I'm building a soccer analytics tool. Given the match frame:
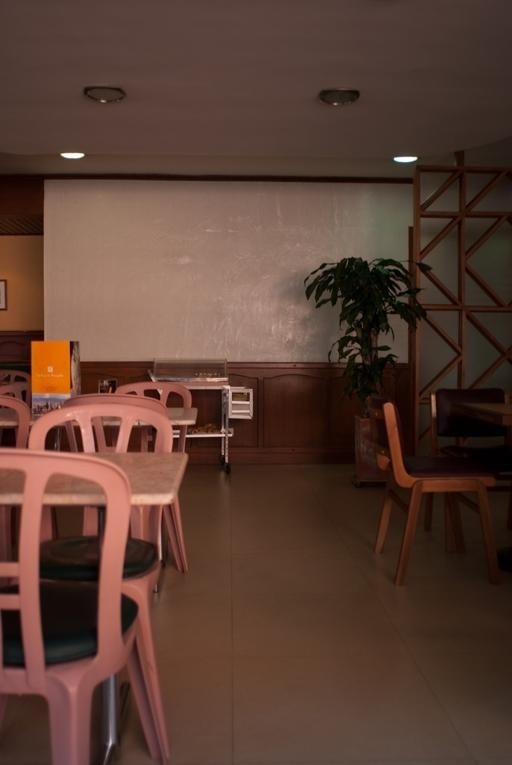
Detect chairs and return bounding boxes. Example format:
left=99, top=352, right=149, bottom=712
left=363, top=397, right=505, bottom=587
left=1, top=370, right=198, bottom=763
left=422, top=382, right=511, bottom=535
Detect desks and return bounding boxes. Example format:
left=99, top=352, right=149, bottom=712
left=435, top=398, right=512, bottom=555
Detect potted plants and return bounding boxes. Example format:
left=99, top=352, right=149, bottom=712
left=301, top=256, right=432, bottom=486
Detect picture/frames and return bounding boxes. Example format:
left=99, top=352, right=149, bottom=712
left=0, top=279, right=8, bottom=312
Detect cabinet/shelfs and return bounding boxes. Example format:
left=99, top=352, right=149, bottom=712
left=145, top=368, right=235, bottom=476
